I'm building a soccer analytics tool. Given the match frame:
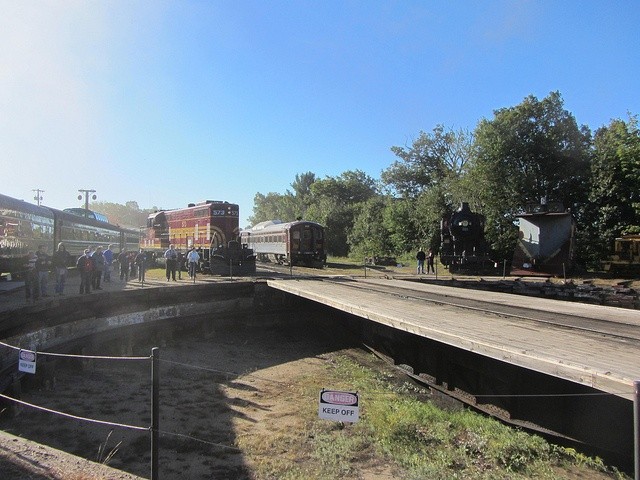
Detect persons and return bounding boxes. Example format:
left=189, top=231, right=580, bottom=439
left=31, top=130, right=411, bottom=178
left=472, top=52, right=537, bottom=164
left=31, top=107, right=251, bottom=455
left=53, top=242, right=72, bottom=296
left=136, top=249, right=147, bottom=282
left=186, top=245, right=201, bottom=280
left=426, top=248, right=435, bottom=274
left=104, top=244, right=113, bottom=283
left=36, top=245, right=52, bottom=303
left=77, top=250, right=96, bottom=294
left=22, top=249, right=41, bottom=302
left=164, top=245, right=177, bottom=281
left=117, top=247, right=129, bottom=282
left=416, top=247, right=426, bottom=274
left=172, top=247, right=183, bottom=280
left=91, top=247, right=104, bottom=291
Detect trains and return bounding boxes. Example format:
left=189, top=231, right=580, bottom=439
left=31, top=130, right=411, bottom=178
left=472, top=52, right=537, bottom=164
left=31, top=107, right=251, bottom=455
left=439, top=201, right=485, bottom=272
left=138, top=200, right=257, bottom=275
left=0, top=193, right=140, bottom=276
left=240, top=219, right=328, bottom=268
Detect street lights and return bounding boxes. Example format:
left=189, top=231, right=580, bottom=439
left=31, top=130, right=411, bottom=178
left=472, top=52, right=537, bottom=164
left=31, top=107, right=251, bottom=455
left=78, top=189, right=97, bottom=218
left=32, top=189, right=45, bottom=206
